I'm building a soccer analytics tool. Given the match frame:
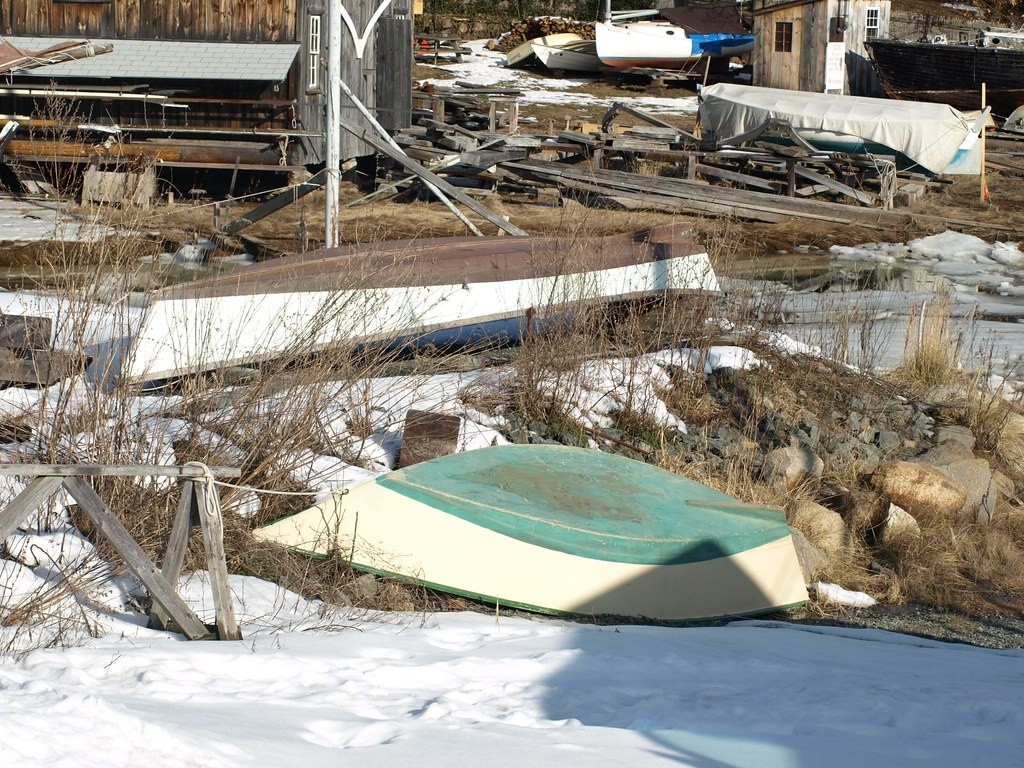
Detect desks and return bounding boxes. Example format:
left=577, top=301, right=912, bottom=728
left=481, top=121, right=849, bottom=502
left=414, top=33, right=463, bottom=65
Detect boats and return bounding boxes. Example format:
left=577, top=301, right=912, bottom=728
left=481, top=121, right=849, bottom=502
left=702, top=81, right=983, bottom=174
left=246, top=439, right=814, bottom=627
left=507, top=19, right=756, bottom=73
left=863, top=37, right=1024, bottom=109
left=112, top=222, right=727, bottom=386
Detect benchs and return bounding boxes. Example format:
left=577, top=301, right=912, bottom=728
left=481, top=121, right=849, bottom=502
left=429, top=47, right=473, bottom=54
left=413, top=48, right=440, bottom=54
